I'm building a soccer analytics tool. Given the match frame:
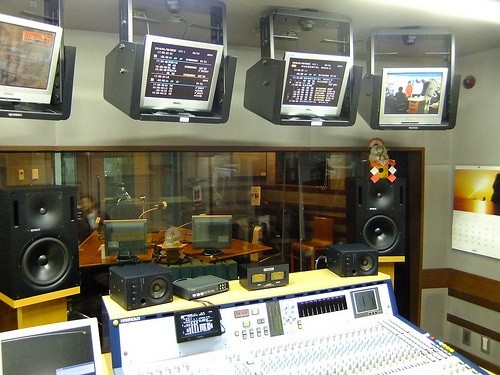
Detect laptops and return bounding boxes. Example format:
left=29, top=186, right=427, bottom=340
left=0, top=317, right=105, bottom=375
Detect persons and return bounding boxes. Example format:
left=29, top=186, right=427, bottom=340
left=405, top=80, right=413, bottom=98
left=396, top=86, right=408, bottom=111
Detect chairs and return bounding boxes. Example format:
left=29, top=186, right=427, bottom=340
left=291, top=217, right=334, bottom=271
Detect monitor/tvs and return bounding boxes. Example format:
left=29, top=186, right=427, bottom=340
left=103, top=218, right=147, bottom=262
left=0, top=13, right=63, bottom=103
left=192, top=214, right=232, bottom=255
left=378, top=67, right=449, bottom=124
left=139, top=33, right=224, bottom=112
left=279, top=51, right=351, bottom=117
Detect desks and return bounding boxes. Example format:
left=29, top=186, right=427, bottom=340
left=79, top=220, right=273, bottom=269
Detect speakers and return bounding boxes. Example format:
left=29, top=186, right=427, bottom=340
left=345, top=151, right=406, bottom=255
left=109, top=262, right=174, bottom=311
left=0, top=184, right=80, bottom=301
left=326, top=243, right=378, bottom=277
